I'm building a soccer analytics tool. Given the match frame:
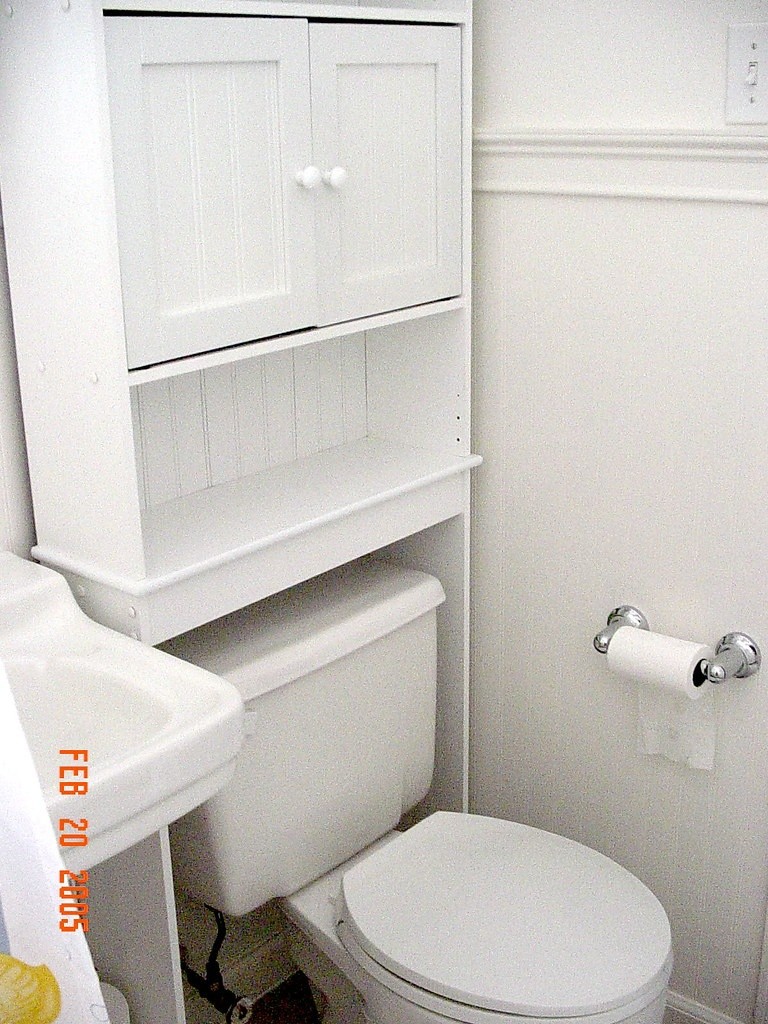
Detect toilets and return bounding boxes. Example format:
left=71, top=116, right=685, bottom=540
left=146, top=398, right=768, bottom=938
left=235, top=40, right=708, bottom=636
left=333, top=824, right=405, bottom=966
left=169, top=557, right=672, bottom=1024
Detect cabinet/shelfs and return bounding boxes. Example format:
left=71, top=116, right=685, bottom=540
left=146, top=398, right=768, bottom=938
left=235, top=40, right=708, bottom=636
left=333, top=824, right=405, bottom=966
left=0, top=0, right=472, bottom=372
left=17, top=300, right=484, bottom=1024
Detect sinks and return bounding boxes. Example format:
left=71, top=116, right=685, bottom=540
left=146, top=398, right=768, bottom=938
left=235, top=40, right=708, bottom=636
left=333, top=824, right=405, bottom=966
left=0, top=557, right=243, bottom=885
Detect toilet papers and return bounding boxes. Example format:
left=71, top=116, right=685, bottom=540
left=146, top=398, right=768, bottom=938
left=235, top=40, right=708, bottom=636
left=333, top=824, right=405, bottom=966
left=606, top=623, right=712, bottom=702
